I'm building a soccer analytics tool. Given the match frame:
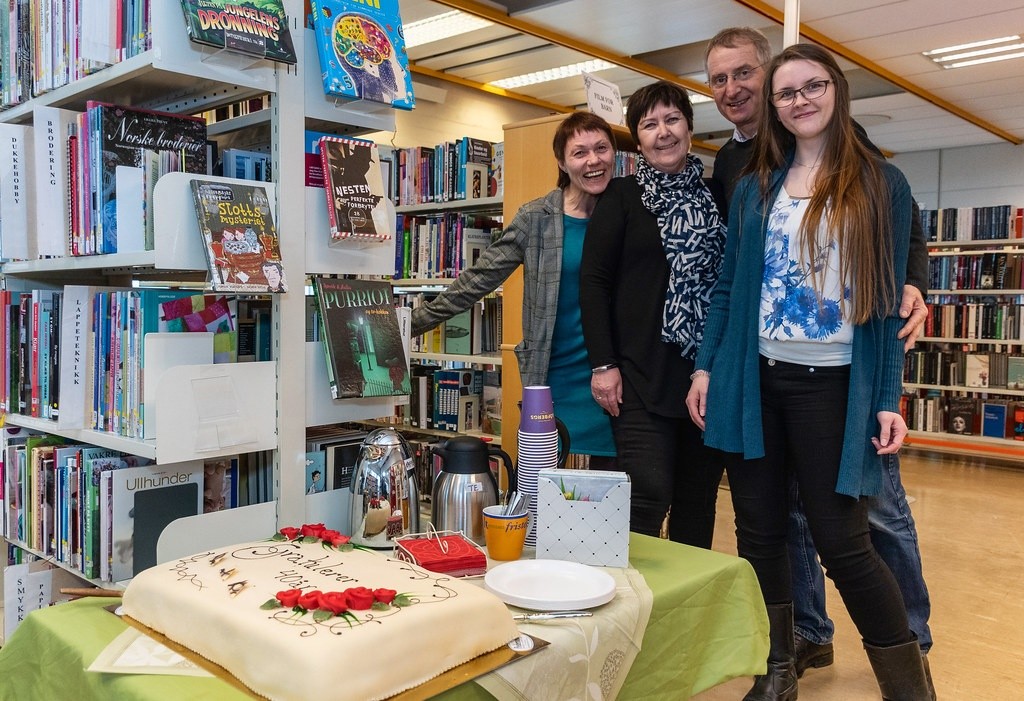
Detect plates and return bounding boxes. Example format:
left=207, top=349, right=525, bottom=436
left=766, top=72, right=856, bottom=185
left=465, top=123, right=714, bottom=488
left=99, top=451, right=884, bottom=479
left=484, top=559, right=617, bottom=611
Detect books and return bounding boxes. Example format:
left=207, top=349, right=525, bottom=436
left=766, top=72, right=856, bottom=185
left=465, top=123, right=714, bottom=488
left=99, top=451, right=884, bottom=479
left=0, top=0, right=516, bottom=657
left=886, top=198, right=1023, bottom=450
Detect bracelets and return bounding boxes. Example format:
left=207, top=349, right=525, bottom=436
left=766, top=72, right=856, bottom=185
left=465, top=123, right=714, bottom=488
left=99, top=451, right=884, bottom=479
left=592, top=364, right=617, bottom=373
left=690, top=370, right=711, bottom=380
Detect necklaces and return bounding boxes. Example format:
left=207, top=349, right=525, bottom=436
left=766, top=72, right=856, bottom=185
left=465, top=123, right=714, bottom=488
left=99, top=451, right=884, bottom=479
left=793, top=160, right=824, bottom=169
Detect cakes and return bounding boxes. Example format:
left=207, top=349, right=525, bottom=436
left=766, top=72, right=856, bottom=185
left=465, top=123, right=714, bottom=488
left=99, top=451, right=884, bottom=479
left=120, top=522, right=521, bottom=701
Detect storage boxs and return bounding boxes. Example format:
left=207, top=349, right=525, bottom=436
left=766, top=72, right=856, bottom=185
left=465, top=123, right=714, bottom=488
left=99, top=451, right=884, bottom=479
left=431, top=366, right=483, bottom=434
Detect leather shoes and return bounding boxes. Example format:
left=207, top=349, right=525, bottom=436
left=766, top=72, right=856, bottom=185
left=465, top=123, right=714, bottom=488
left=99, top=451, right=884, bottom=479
left=793, top=632, right=834, bottom=678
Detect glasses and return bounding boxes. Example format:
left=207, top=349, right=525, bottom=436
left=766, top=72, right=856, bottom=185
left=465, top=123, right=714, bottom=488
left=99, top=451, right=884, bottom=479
left=704, top=61, right=770, bottom=89
left=768, top=79, right=835, bottom=108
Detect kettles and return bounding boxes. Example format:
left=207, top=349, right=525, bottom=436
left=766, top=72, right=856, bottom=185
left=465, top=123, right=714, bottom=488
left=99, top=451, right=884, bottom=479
left=428, top=436, right=514, bottom=547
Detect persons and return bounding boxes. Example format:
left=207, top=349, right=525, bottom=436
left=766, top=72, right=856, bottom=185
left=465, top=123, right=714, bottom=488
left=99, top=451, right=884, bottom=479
left=685, top=43, right=940, bottom=701
left=705, top=27, right=930, bottom=682
left=408, top=109, right=624, bottom=492
left=580, top=81, right=734, bottom=552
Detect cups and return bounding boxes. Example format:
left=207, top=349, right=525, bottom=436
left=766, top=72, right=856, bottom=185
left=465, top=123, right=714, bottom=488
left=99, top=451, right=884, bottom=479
left=518, top=385, right=558, bottom=548
left=483, top=504, right=534, bottom=561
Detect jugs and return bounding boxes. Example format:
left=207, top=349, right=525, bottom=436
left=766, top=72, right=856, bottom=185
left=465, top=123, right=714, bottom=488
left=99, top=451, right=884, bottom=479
left=346, top=427, right=420, bottom=550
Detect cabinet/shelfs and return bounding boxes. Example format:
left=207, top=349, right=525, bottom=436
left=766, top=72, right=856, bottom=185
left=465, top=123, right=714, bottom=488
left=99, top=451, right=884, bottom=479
left=902, top=204, right=1023, bottom=466
left=351, top=193, right=503, bottom=518
left=0, top=51, right=277, bottom=591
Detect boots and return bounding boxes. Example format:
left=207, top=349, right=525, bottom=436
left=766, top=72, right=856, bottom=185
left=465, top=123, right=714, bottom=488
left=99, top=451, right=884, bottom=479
left=860, top=629, right=932, bottom=701
left=742, top=600, right=798, bottom=701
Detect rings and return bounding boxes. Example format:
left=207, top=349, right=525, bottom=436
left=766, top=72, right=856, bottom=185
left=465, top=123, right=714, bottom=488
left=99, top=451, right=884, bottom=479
left=596, top=396, right=602, bottom=399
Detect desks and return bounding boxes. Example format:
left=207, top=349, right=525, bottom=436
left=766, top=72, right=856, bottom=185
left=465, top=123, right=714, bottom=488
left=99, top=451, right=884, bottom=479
left=0, top=510, right=772, bottom=701
